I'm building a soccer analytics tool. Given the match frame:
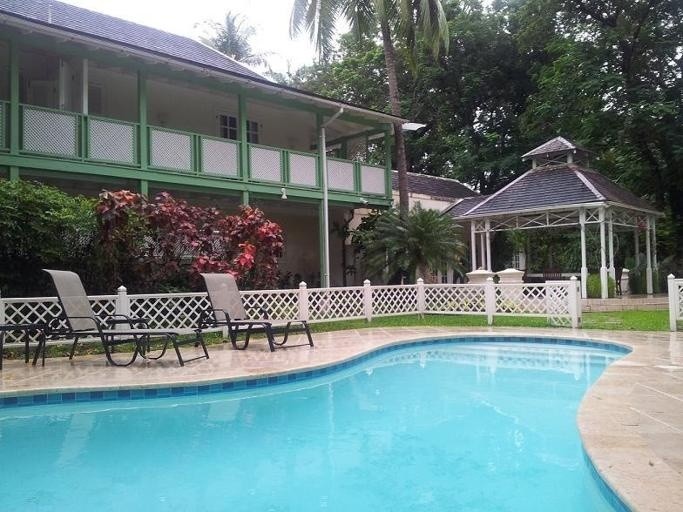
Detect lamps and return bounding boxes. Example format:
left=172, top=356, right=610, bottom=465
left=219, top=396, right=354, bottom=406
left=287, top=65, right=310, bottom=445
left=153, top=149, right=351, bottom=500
left=359, top=198, right=369, bottom=206
left=281, top=188, right=288, bottom=200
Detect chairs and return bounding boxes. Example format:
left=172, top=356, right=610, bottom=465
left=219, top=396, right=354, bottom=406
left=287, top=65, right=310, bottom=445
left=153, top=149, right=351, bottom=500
left=543, top=268, right=561, bottom=295
left=40, top=268, right=209, bottom=367
left=195, top=272, right=314, bottom=352
left=615, top=267, right=622, bottom=295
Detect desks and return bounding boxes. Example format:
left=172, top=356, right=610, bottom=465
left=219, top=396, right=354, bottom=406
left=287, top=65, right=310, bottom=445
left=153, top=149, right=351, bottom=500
left=106, top=318, right=152, bottom=356
left=0, top=323, right=46, bottom=371
